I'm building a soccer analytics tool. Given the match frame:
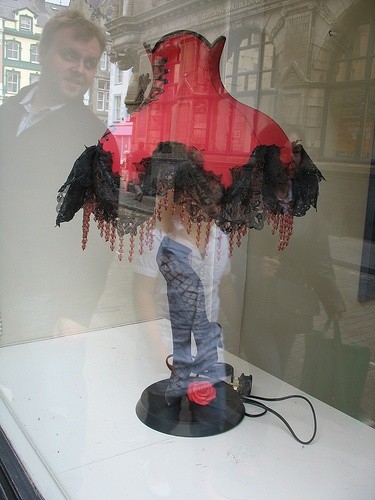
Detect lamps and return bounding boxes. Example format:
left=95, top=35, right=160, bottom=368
left=53, top=28, right=326, bottom=441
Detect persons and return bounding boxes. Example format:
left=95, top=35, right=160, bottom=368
left=218, top=120, right=346, bottom=382
left=0, top=13, right=119, bottom=345
left=135, top=160, right=230, bottom=436
left=55, top=29, right=319, bottom=408
